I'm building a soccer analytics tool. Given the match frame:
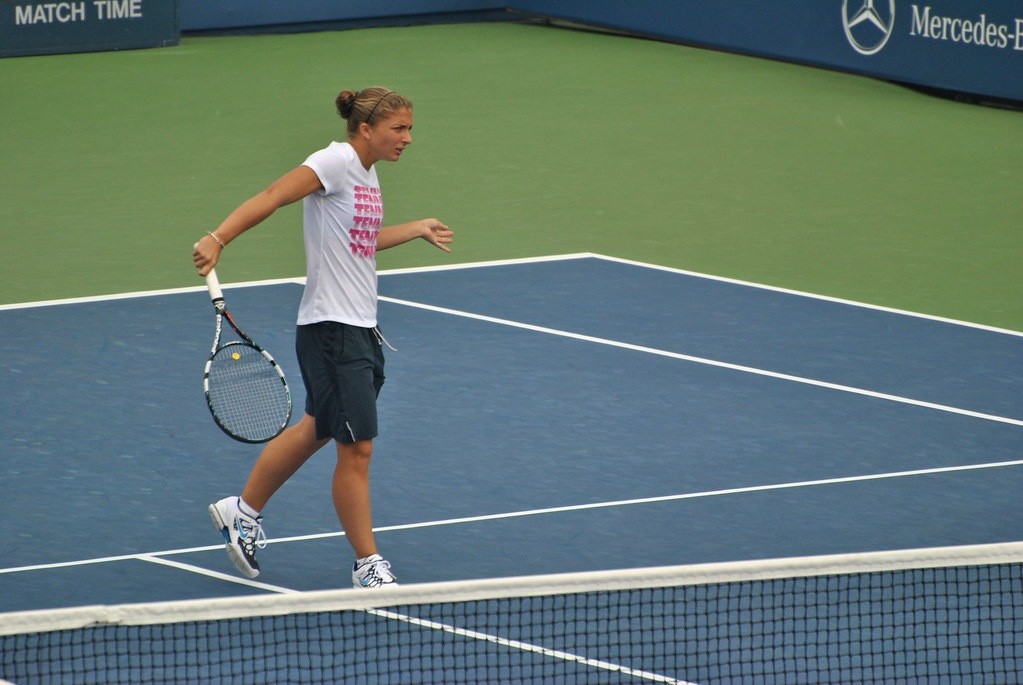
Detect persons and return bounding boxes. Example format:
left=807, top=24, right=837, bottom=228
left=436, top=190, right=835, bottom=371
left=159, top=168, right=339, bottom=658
left=192, top=87, right=455, bottom=590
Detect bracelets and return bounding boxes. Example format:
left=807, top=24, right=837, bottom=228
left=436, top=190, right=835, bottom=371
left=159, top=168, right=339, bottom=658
left=209, top=232, right=225, bottom=248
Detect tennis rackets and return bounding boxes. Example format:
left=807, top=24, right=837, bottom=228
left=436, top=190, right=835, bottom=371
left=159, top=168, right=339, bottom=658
left=193, top=241, right=293, bottom=444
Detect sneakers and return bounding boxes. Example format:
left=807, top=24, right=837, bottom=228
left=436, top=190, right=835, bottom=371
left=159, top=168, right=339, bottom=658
left=351, top=558, right=398, bottom=588
left=210, top=494, right=268, bottom=578
